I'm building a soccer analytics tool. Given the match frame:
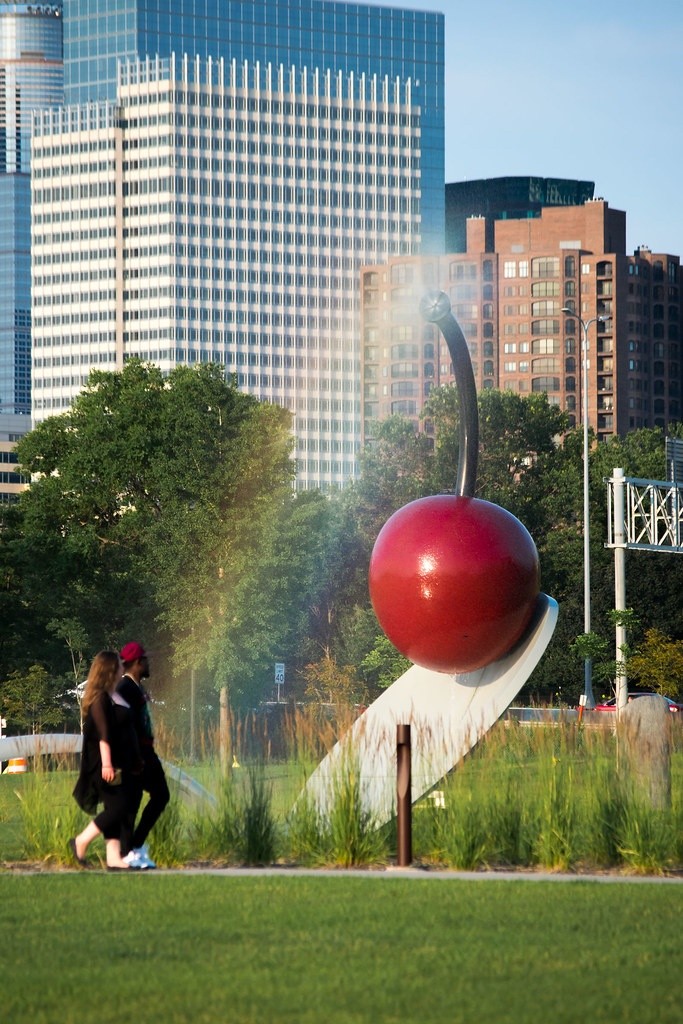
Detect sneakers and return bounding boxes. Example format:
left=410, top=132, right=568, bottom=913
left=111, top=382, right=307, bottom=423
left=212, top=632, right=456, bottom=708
left=123, top=844, right=156, bottom=869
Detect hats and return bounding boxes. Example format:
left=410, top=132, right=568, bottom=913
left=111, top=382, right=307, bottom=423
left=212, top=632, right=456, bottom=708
left=119, top=641, right=145, bottom=663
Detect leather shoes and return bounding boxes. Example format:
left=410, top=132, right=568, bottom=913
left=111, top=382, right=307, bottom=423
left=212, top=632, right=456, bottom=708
left=106, top=864, right=136, bottom=872
left=70, top=839, right=87, bottom=865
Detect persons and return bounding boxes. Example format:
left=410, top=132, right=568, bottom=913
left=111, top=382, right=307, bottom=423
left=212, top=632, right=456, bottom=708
left=111, top=642, right=170, bottom=869
left=65, top=650, right=143, bottom=872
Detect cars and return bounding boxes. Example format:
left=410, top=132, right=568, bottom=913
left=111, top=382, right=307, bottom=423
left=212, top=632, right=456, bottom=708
left=594, top=692, right=683, bottom=715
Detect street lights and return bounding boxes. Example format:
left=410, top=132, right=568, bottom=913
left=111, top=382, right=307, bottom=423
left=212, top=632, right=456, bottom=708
left=562, top=306, right=609, bottom=704
